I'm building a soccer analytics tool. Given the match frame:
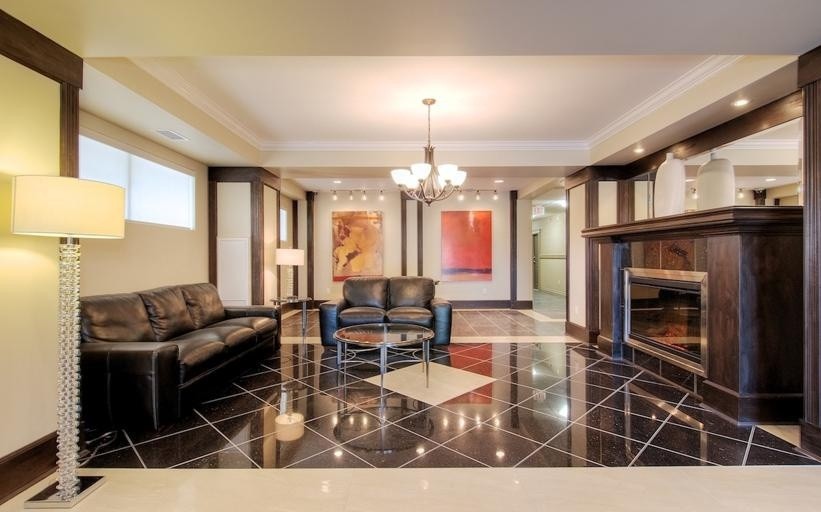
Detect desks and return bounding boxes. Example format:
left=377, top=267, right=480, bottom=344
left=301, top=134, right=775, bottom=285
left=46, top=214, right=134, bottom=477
left=270, top=296, right=313, bottom=331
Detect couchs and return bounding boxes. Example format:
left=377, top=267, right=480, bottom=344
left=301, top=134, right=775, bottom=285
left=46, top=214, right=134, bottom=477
left=319, top=276, right=454, bottom=348
left=79, top=282, right=282, bottom=435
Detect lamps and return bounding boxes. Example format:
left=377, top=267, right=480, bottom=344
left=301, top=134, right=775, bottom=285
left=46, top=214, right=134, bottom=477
left=9, top=173, right=127, bottom=509
left=276, top=248, right=306, bottom=300
left=274, top=385, right=307, bottom=443
left=390, top=98, right=467, bottom=209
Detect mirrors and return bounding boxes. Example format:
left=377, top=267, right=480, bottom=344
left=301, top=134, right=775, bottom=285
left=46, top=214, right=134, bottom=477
left=208, top=166, right=282, bottom=306
left=565, top=165, right=621, bottom=345
left=626, top=114, right=805, bottom=223
